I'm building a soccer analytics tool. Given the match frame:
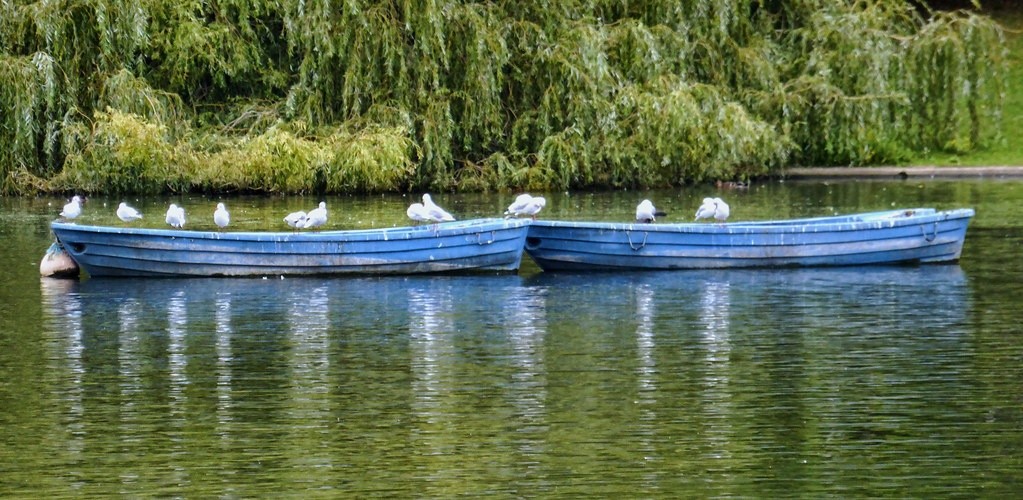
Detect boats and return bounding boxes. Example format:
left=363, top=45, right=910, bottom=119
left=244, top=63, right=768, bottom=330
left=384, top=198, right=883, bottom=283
left=51, top=216, right=529, bottom=277
left=521, top=205, right=978, bottom=271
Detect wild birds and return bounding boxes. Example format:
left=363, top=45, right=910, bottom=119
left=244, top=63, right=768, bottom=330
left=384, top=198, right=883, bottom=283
left=636, top=199, right=667, bottom=223
left=165, top=204, right=185, bottom=230
left=116, top=202, right=142, bottom=223
left=283, top=201, right=328, bottom=234
left=213, top=203, right=229, bottom=233
left=406, top=193, right=456, bottom=230
left=694, top=197, right=730, bottom=223
left=58, top=195, right=85, bottom=219
left=504, top=194, right=546, bottom=221
